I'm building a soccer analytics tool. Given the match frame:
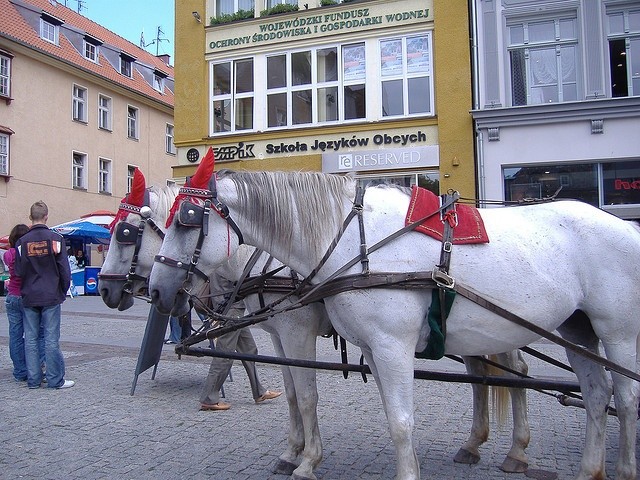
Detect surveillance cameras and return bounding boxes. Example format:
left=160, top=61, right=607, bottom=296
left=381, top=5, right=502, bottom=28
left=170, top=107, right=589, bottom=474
left=192, top=11, right=201, bottom=23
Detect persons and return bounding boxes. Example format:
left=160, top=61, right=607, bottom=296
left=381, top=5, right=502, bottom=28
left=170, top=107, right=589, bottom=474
left=3, top=224, right=49, bottom=383
left=198, top=272, right=282, bottom=410
left=14, top=201, right=75, bottom=388
left=75, top=250, right=88, bottom=267
left=67, top=248, right=79, bottom=270
left=164, top=314, right=191, bottom=345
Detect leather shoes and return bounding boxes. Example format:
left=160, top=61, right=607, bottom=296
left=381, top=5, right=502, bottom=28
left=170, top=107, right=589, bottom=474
left=201, top=401, right=232, bottom=410
left=254, top=391, right=283, bottom=403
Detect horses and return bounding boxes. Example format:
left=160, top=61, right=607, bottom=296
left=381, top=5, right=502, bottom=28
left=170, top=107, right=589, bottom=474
left=147, top=144, right=640, bottom=479
left=92, top=168, right=535, bottom=474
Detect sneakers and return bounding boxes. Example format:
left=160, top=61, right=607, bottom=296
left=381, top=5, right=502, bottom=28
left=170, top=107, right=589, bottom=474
left=48, top=380, right=75, bottom=389
left=165, top=339, right=178, bottom=344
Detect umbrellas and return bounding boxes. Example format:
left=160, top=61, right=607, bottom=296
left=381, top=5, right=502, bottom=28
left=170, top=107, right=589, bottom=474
left=75, top=216, right=116, bottom=261
left=0, top=236, right=9, bottom=243
left=80, top=210, right=117, bottom=219
left=49, top=218, right=112, bottom=252
